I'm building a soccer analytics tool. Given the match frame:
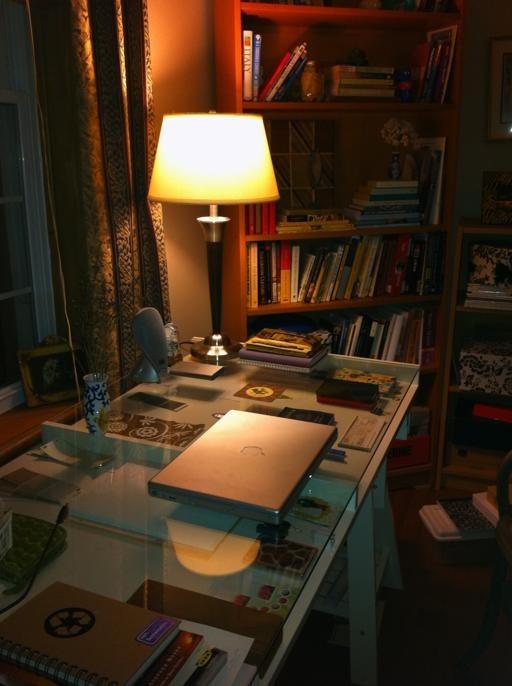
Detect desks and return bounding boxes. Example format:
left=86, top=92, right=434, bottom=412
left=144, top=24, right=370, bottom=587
left=0, top=342, right=421, bottom=685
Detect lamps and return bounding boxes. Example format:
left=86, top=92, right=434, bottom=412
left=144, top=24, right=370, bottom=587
left=147, top=109, right=280, bottom=365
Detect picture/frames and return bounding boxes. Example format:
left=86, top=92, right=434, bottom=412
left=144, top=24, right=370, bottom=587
left=17, top=342, right=91, bottom=408
left=487, top=36, right=512, bottom=142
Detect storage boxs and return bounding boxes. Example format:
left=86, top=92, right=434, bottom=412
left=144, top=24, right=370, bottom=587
left=476, top=170, right=512, bottom=224
left=447, top=391, right=511, bottom=451
left=459, top=334, right=512, bottom=397
left=470, top=244, right=510, bottom=289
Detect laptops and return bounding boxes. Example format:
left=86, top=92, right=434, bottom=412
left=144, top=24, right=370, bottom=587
left=149, top=410, right=338, bottom=527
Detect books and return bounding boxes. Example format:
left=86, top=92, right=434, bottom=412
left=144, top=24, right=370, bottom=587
left=246, top=234, right=420, bottom=307
left=243, top=30, right=452, bottom=105
left=0, top=467, right=81, bottom=505
left=360, top=0, right=449, bottom=13
left=186, top=648, right=228, bottom=686
left=464, top=245, right=512, bottom=311
left=169, top=360, right=225, bottom=381
left=240, top=310, right=409, bottom=373
left=1, top=579, right=182, bottom=686
left=246, top=180, right=423, bottom=236
left=261, top=0, right=332, bottom=7
left=1, top=626, right=207, bottom=686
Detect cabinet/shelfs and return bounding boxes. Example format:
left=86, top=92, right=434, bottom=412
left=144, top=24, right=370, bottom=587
left=212, top=1, right=466, bottom=491
left=433, top=217, right=512, bottom=496
